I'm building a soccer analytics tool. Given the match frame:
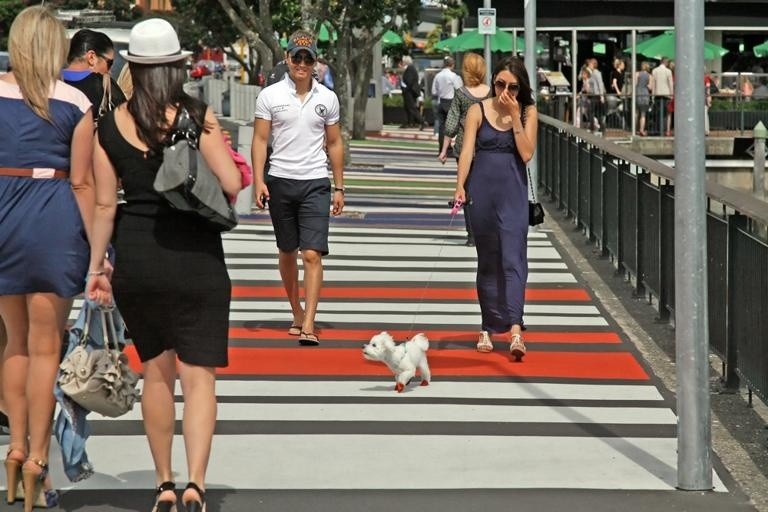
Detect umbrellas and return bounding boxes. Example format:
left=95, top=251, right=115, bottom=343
left=432, top=27, right=544, bottom=54
left=753, top=40, right=768, bottom=58
left=279, top=23, right=403, bottom=49
left=623, top=31, right=730, bottom=62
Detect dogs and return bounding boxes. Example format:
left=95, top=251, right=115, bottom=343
left=360, top=331, right=432, bottom=393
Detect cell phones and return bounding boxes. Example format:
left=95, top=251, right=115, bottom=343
left=262, top=197, right=266, bottom=205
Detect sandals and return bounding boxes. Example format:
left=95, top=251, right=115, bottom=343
left=509, top=334, right=526, bottom=361
left=476, top=330, right=494, bottom=353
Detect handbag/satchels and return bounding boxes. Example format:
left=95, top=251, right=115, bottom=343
left=527, top=200, right=545, bottom=226
left=58, top=305, right=141, bottom=418
left=153, top=103, right=238, bottom=232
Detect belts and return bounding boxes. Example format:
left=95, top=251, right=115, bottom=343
left=0, top=168, right=69, bottom=178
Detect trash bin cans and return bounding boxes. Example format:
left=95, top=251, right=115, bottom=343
left=347, top=80, right=383, bottom=135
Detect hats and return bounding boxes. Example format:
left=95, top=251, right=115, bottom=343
left=118, top=17, right=195, bottom=65
left=287, top=30, right=318, bottom=61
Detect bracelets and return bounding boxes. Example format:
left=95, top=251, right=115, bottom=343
left=335, top=188, right=344, bottom=193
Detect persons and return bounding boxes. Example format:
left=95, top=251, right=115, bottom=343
left=453, top=57, right=538, bottom=362
left=704, top=66, right=754, bottom=137
left=431, top=53, right=495, bottom=247
left=251, top=29, right=346, bottom=348
left=382, top=55, right=428, bottom=128
left=578, top=54, right=676, bottom=137
left=0, top=4, right=242, bottom=512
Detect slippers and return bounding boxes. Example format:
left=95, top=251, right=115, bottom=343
left=287, top=325, right=301, bottom=336
left=299, top=332, right=320, bottom=345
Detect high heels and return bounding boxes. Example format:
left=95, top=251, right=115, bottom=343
left=22, top=457, right=59, bottom=512
left=182, top=483, right=207, bottom=512
left=150, top=481, right=180, bottom=512
left=5, top=445, right=28, bottom=503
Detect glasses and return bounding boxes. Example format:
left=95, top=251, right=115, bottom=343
left=290, top=54, right=315, bottom=66
left=100, top=54, right=114, bottom=70
left=494, top=81, right=519, bottom=93
